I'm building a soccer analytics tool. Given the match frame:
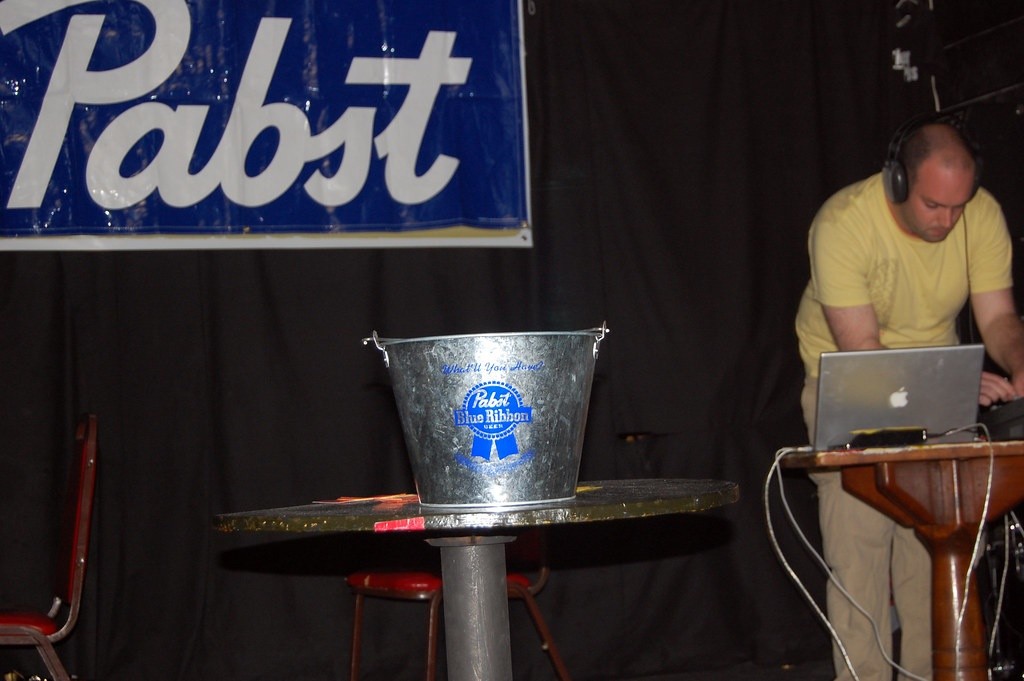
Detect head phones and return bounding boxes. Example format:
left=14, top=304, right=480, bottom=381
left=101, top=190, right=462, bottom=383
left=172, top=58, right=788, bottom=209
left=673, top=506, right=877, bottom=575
left=885, top=111, right=981, bottom=203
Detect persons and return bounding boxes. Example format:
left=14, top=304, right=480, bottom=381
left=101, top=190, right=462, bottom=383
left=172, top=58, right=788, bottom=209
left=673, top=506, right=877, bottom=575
left=794, top=120, right=1024, bottom=681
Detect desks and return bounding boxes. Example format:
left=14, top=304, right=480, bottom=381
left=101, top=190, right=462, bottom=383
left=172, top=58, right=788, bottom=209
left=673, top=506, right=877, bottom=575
left=214, top=479, right=740, bottom=681
left=782, top=440, right=1024, bottom=680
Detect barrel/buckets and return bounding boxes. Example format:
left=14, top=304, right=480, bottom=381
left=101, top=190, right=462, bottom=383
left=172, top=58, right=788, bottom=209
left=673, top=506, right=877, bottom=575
left=361, top=322, right=612, bottom=509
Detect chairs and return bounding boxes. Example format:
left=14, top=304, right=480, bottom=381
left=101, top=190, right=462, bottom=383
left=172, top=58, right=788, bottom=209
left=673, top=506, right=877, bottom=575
left=347, top=523, right=571, bottom=681
left=0, top=411, right=98, bottom=680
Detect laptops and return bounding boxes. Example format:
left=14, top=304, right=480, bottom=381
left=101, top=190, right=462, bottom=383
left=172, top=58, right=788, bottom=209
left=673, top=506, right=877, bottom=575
left=811, top=343, right=985, bottom=452
